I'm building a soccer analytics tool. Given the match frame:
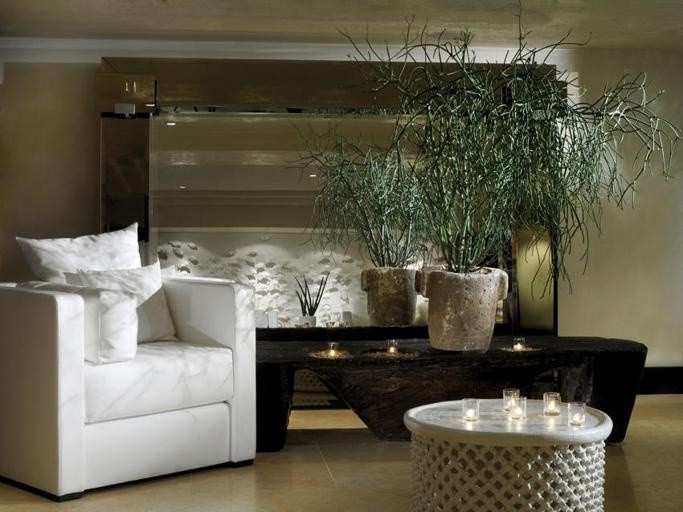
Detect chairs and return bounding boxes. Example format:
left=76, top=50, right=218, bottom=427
left=0, top=266, right=259, bottom=503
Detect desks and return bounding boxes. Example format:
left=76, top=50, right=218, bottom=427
left=256, top=336, right=648, bottom=454
left=399, top=396, right=613, bottom=510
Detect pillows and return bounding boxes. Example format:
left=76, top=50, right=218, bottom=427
left=16, top=222, right=142, bottom=297
left=65, top=258, right=183, bottom=343
left=16, top=280, right=137, bottom=363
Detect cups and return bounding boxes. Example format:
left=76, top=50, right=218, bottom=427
left=512, top=337, right=525, bottom=350
left=542, top=392, right=562, bottom=415
left=462, top=398, right=481, bottom=421
left=268, top=310, right=277, bottom=328
left=501, top=387, right=527, bottom=420
left=566, top=401, right=586, bottom=426
left=387, top=339, right=400, bottom=354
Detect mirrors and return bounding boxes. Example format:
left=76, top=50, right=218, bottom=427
left=146, top=105, right=522, bottom=328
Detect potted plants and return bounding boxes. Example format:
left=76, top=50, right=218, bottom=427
left=297, top=118, right=430, bottom=325
left=304, top=16, right=682, bottom=350
left=291, top=264, right=329, bottom=327
